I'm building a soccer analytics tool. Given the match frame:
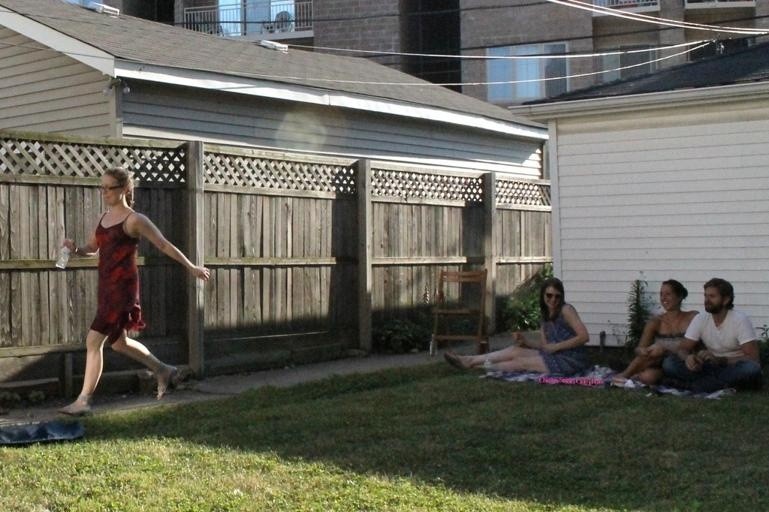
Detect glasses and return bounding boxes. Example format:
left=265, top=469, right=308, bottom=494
left=99, top=185, right=123, bottom=192
left=546, top=293, right=561, bottom=299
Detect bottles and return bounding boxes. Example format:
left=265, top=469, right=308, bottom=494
left=592, top=364, right=605, bottom=393
left=55, top=246, right=70, bottom=269
left=430, top=334, right=438, bottom=359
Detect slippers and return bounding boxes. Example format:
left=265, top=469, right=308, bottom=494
left=444, top=352, right=471, bottom=370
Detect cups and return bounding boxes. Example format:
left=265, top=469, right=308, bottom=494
left=480, top=341, right=489, bottom=355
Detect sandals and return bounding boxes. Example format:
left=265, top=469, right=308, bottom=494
left=58, top=395, right=91, bottom=417
left=155, top=362, right=178, bottom=401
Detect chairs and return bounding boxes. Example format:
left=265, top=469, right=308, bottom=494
left=429, top=269, right=488, bottom=356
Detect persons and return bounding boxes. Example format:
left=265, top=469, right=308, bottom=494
left=659, top=277, right=764, bottom=395
left=608, top=277, right=699, bottom=388
left=59, top=166, right=210, bottom=415
left=445, top=277, right=594, bottom=377
